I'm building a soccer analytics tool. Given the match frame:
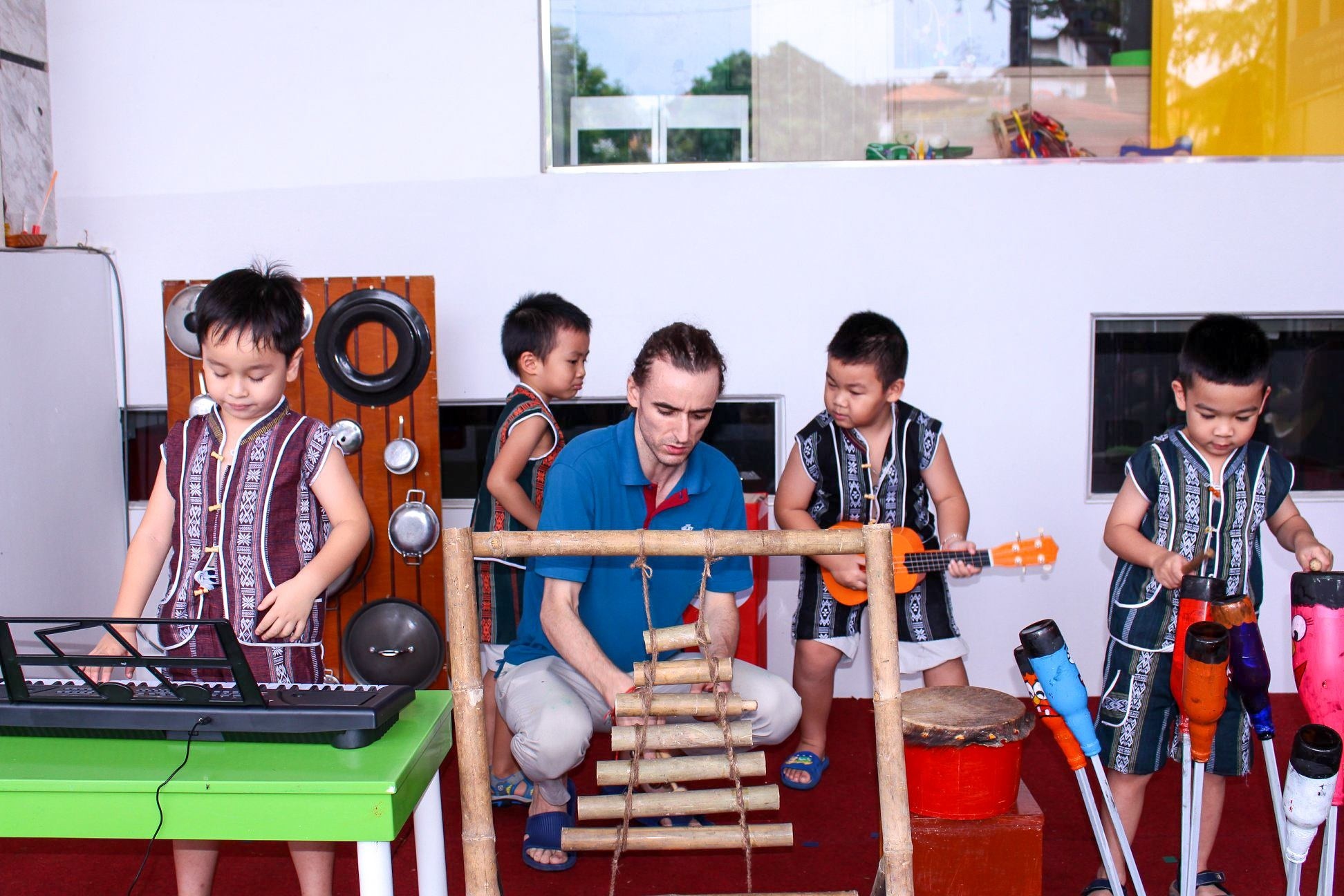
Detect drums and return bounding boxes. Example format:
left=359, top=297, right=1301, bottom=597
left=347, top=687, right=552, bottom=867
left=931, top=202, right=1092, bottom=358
left=902, top=685, right=1036, bottom=819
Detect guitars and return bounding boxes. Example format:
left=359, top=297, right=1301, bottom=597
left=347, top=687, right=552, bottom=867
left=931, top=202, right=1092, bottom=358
left=820, top=520, right=1059, bottom=606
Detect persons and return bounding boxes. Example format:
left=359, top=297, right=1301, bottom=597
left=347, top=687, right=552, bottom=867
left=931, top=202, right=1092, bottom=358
left=76, top=267, right=372, bottom=895
left=1077, top=312, right=1333, bottom=896
left=493, top=321, right=803, bottom=872
left=774, top=310, right=985, bottom=789
left=467, top=292, right=594, bottom=806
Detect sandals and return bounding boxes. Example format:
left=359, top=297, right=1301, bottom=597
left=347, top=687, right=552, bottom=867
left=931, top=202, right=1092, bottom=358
left=1081, top=879, right=1128, bottom=896
left=489, top=770, right=535, bottom=807
left=1168, top=870, right=1235, bottom=896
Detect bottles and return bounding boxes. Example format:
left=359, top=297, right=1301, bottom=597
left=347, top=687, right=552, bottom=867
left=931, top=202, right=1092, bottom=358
left=1290, top=571, right=1344, bottom=806
left=1019, top=618, right=1102, bottom=757
left=1014, top=644, right=1088, bottom=771
left=1182, top=623, right=1229, bottom=763
left=1212, top=596, right=1274, bottom=742
left=1282, top=724, right=1343, bottom=866
left=1170, top=575, right=1227, bottom=733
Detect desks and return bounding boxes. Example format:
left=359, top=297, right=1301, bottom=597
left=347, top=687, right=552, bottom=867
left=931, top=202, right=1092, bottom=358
left=0, top=689, right=456, bottom=896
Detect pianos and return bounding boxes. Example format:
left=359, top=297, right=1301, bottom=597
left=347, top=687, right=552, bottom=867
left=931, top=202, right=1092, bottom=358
left=0, top=677, right=416, bottom=751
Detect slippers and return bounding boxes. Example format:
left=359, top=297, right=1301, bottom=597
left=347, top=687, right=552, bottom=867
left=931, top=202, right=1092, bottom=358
left=780, top=751, right=829, bottom=790
left=521, top=779, right=577, bottom=871
left=604, top=785, right=714, bottom=827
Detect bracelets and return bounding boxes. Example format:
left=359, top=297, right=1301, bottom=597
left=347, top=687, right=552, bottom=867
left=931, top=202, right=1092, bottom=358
left=942, top=534, right=962, bottom=542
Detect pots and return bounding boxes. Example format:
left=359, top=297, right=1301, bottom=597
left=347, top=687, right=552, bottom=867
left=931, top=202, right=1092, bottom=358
left=383, top=414, right=420, bottom=475
left=387, top=489, right=439, bottom=565
